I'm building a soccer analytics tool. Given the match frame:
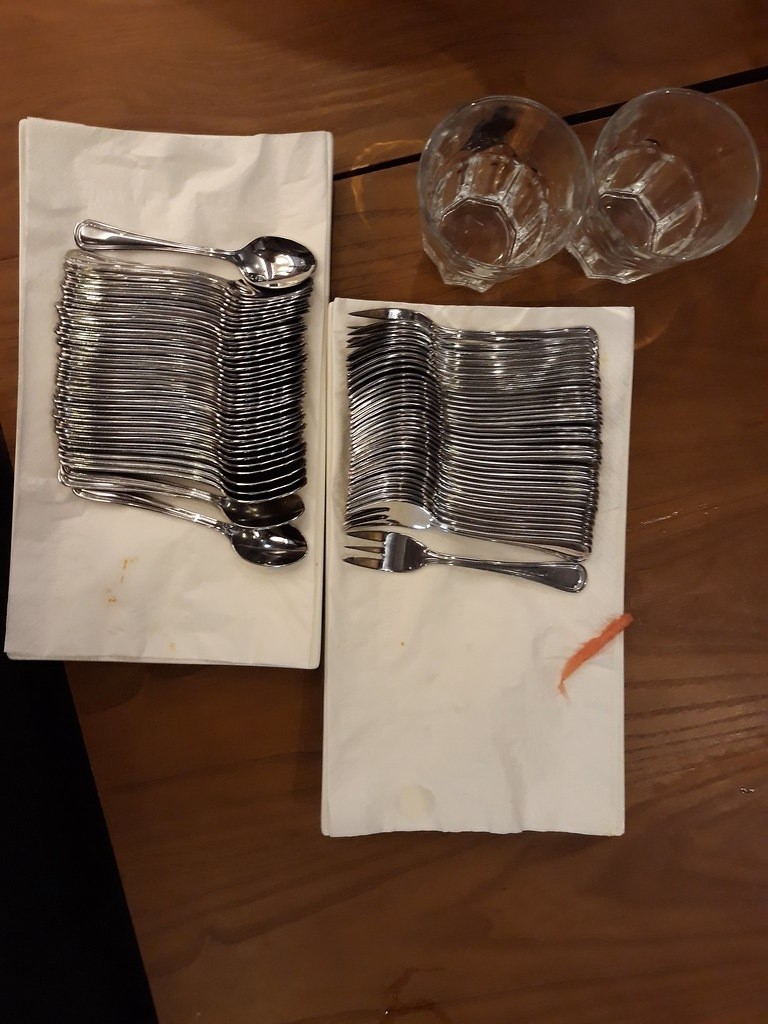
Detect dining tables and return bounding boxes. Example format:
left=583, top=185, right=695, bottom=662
left=0, top=0, right=768, bottom=1017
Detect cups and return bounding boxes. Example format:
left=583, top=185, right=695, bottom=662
left=420, top=92, right=590, bottom=295
left=564, top=87, right=762, bottom=285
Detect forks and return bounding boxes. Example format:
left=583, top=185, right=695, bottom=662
left=330, top=298, right=609, bottom=601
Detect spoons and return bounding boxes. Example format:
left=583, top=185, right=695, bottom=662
left=50, top=217, right=314, bottom=569
